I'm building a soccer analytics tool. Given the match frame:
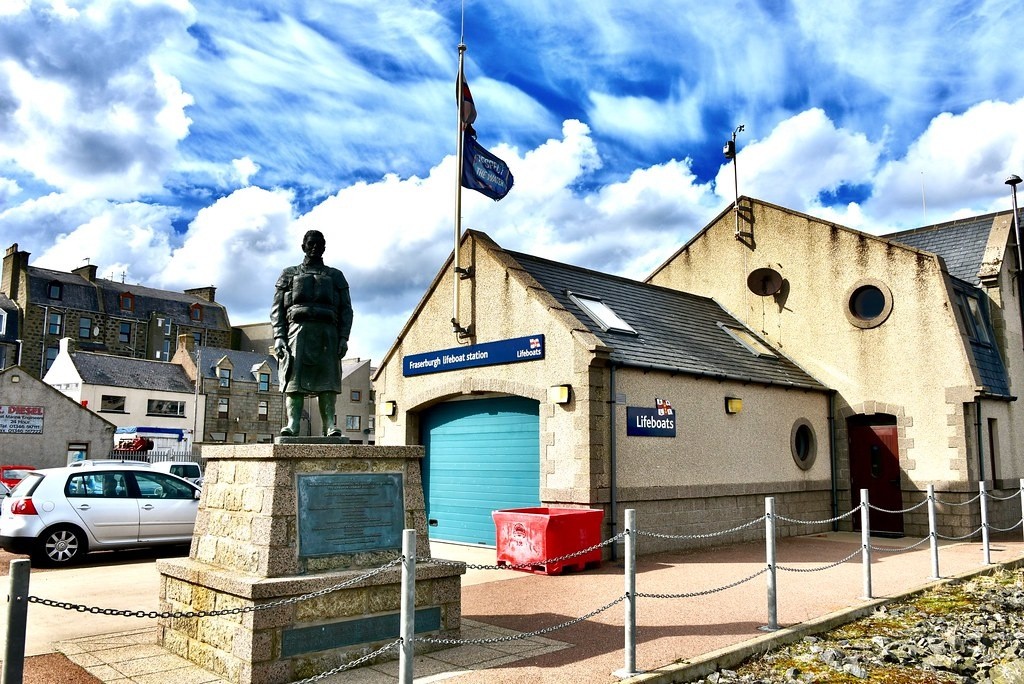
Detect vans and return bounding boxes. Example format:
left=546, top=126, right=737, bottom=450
left=64, top=457, right=203, bottom=497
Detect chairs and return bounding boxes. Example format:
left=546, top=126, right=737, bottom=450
left=175, top=469, right=181, bottom=476
left=118, top=478, right=126, bottom=497
left=103, top=479, right=118, bottom=497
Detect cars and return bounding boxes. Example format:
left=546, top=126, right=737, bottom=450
left=0, top=465, right=202, bottom=568
left=0, top=465, right=36, bottom=488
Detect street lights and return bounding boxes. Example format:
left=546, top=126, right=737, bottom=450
left=1003, top=172, right=1024, bottom=272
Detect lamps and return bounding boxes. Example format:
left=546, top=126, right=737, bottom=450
left=11, top=375, right=20, bottom=383
left=380, top=401, right=397, bottom=417
left=724, top=396, right=743, bottom=415
left=550, top=384, right=572, bottom=406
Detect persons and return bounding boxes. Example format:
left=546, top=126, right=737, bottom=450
left=269, top=230, right=354, bottom=437
